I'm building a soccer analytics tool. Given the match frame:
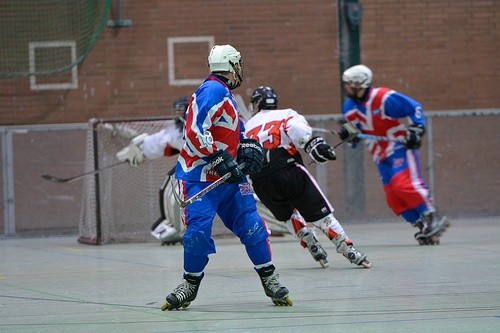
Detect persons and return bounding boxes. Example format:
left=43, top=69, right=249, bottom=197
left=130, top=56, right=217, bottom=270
left=116, top=98, right=190, bottom=241
left=338, top=65, right=447, bottom=239
left=242, top=85, right=364, bottom=264
left=165, top=45, right=289, bottom=306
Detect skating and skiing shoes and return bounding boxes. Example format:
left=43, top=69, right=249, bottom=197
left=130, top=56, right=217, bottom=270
left=336, top=238, right=372, bottom=268
left=160, top=272, right=205, bottom=310
left=303, top=232, right=330, bottom=268
left=412, top=209, right=450, bottom=245
left=253, top=265, right=294, bottom=307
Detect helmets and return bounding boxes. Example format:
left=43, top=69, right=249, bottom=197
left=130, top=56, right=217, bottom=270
left=342, top=64, right=375, bottom=89
left=171, top=96, right=190, bottom=121
left=206, top=44, right=245, bottom=90
left=251, top=85, right=279, bottom=111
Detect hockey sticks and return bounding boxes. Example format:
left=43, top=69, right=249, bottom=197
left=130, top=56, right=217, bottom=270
left=305, top=119, right=360, bottom=167
left=312, top=126, right=397, bottom=142
left=171, top=162, right=246, bottom=208
left=41, top=159, right=129, bottom=183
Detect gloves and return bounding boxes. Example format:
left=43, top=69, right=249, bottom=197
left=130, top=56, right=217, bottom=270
left=237, top=138, right=266, bottom=174
left=404, top=124, right=426, bottom=151
left=303, top=135, right=337, bottom=165
left=209, top=149, right=247, bottom=184
left=335, top=118, right=358, bottom=142
